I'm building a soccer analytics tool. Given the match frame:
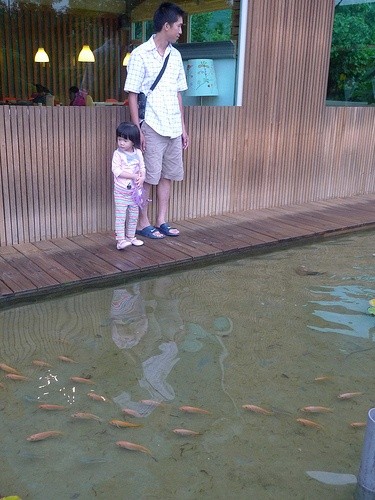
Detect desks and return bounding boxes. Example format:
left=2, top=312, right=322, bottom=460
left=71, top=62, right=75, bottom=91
left=92, top=101, right=125, bottom=106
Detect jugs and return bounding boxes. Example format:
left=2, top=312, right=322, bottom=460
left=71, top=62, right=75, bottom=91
left=45, top=93, right=55, bottom=106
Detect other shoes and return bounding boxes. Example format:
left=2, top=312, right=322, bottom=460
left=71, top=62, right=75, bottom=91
left=126, top=237, right=144, bottom=246
left=116, top=240, right=132, bottom=250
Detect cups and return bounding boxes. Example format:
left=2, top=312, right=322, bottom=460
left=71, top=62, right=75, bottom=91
left=38, top=103, right=42, bottom=106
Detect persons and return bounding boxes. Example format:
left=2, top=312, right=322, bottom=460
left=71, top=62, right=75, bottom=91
left=109, top=283, right=148, bottom=381
left=124, top=3, right=188, bottom=240
left=33, top=85, right=95, bottom=106
left=111, top=121, right=146, bottom=250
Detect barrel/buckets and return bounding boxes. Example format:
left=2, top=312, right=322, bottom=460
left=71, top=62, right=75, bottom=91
left=356, top=407, right=375, bottom=500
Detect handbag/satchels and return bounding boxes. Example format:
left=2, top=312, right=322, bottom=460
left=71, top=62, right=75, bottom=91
left=137, top=92, right=147, bottom=119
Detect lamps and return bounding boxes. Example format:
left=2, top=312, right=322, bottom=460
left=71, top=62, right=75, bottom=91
left=34, top=47, right=49, bottom=63
left=122, top=50, right=132, bottom=66
left=186, top=57, right=221, bottom=107
left=77, top=0, right=96, bottom=63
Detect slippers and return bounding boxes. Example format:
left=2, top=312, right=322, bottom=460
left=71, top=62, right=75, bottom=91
left=136, top=225, right=165, bottom=239
left=152, top=223, right=180, bottom=236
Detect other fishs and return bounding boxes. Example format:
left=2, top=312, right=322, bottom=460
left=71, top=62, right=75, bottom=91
left=0, top=354, right=368, bottom=470
